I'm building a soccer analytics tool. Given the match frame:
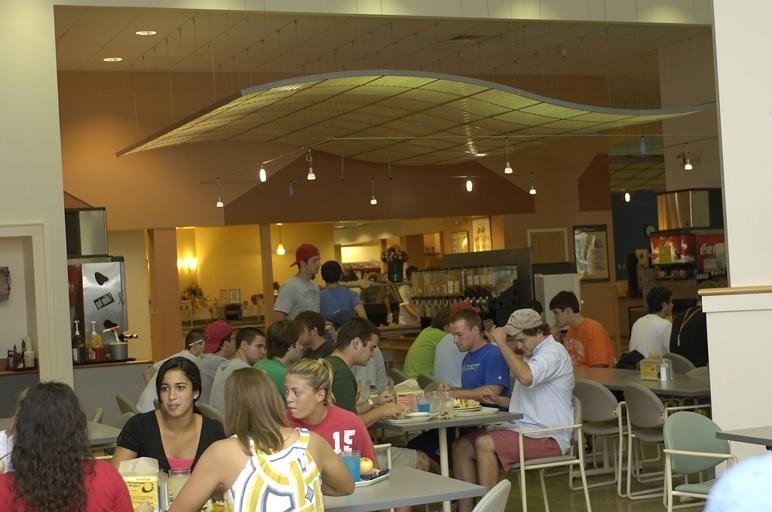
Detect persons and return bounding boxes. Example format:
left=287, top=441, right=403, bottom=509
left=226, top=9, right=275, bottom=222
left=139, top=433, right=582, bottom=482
left=548, top=290, right=616, bottom=454
left=110, top=357, right=228, bottom=473
left=628, top=286, right=674, bottom=358
left=274, top=243, right=321, bottom=327
left=314, top=259, right=370, bottom=325
left=670, top=280, right=722, bottom=419
left=0, top=385, right=33, bottom=474
left=397, top=265, right=422, bottom=326
left=0, top=381, right=155, bottom=511
left=361, top=270, right=396, bottom=326
left=404, top=308, right=510, bottom=512
left=135, top=307, right=468, bottom=512
left=452, top=307, right=575, bottom=512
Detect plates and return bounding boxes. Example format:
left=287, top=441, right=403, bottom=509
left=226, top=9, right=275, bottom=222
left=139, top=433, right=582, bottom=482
left=355, top=468, right=391, bottom=487
left=405, top=413, right=437, bottom=422
left=454, top=406, right=500, bottom=415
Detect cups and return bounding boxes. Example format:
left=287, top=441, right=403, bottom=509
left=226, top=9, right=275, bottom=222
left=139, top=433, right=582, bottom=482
left=339, top=450, right=360, bottom=482
left=416, top=395, right=430, bottom=413
left=442, top=391, right=455, bottom=409
left=168, top=467, right=191, bottom=502
left=428, top=391, right=442, bottom=412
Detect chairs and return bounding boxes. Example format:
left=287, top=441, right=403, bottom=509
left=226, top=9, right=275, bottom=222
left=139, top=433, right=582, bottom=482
left=507, top=395, right=594, bottom=510
left=569, top=381, right=664, bottom=500
left=196, top=402, right=229, bottom=433
left=113, top=413, right=137, bottom=430
left=90, top=406, right=109, bottom=423
left=662, top=409, right=738, bottom=511
left=370, top=442, right=431, bottom=511
left=662, top=350, right=699, bottom=413
left=623, top=382, right=704, bottom=502
left=471, top=477, right=512, bottom=512
left=115, top=392, right=146, bottom=412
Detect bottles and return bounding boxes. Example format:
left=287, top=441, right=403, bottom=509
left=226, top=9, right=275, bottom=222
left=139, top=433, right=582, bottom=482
left=6, top=340, right=26, bottom=370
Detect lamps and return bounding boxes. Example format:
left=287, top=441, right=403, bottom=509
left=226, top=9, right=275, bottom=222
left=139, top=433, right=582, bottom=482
left=368, top=178, right=378, bottom=205
left=677, top=138, right=693, bottom=173
left=637, top=134, right=647, bottom=157
left=502, top=135, right=512, bottom=176
left=305, top=146, right=317, bottom=182
left=276, top=222, right=286, bottom=255
left=259, top=160, right=268, bottom=182
left=286, top=178, right=296, bottom=196
left=465, top=176, right=474, bottom=192
left=623, top=183, right=633, bottom=209
left=527, top=171, right=538, bottom=202
left=215, top=178, right=224, bottom=209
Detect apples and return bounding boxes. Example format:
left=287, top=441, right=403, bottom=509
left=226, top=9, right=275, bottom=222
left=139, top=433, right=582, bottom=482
left=360, top=457, right=373, bottom=476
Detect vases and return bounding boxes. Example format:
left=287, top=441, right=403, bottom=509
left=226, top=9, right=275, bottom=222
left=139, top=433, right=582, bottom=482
left=386, top=263, right=404, bottom=283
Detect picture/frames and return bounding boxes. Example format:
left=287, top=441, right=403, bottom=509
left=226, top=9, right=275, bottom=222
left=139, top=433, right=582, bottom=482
left=228, top=286, right=241, bottom=304
left=449, top=231, right=470, bottom=254
left=572, top=223, right=610, bottom=285
left=471, top=216, right=492, bottom=253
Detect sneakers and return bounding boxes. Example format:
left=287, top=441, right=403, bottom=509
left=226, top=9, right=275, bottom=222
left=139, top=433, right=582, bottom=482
left=584, top=434, right=592, bottom=453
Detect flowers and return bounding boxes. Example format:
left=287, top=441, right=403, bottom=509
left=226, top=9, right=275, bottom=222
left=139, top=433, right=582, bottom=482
left=380, top=242, right=408, bottom=263
left=405, top=265, right=419, bottom=276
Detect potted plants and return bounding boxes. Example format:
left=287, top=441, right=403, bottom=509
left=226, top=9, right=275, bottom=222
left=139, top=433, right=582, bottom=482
left=361, top=282, right=389, bottom=326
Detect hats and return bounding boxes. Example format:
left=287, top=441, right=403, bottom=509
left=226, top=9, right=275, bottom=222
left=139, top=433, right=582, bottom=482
left=203, top=321, right=232, bottom=353
left=503, top=308, right=543, bottom=336
left=327, top=308, right=358, bottom=328
left=450, top=302, right=481, bottom=315
left=699, top=280, right=719, bottom=288
left=289, top=244, right=319, bottom=267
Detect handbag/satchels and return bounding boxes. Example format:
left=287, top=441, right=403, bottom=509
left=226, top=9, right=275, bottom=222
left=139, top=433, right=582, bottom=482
left=611, top=350, right=646, bottom=402
